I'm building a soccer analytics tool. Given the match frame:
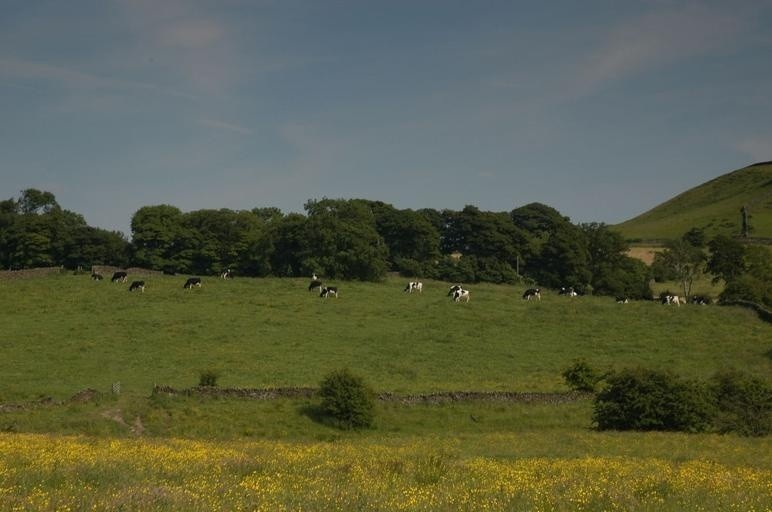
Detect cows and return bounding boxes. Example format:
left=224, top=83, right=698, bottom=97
left=184, top=278, right=201, bottom=289
left=523, top=288, right=541, bottom=302
left=447, top=285, right=470, bottom=303
left=320, top=286, right=338, bottom=298
left=111, top=272, right=128, bottom=283
left=558, top=286, right=583, bottom=297
left=404, top=282, right=423, bottom=293
left=91, top=274, right=103, bottom=280
left=220, top=269, right=231, bottom=280
left=129, top=280, right=144, bottom=292
left=662, top=294, right=709, bottom=307
left=308, top=275, right=322, bottom=292
left=616, top=296, right=628, bottom=304
